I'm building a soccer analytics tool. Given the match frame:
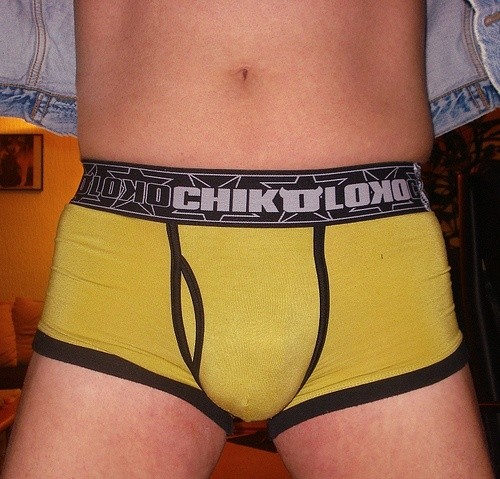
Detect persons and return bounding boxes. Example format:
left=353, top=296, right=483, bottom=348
left=3, top=2, right=500, bottom=476
